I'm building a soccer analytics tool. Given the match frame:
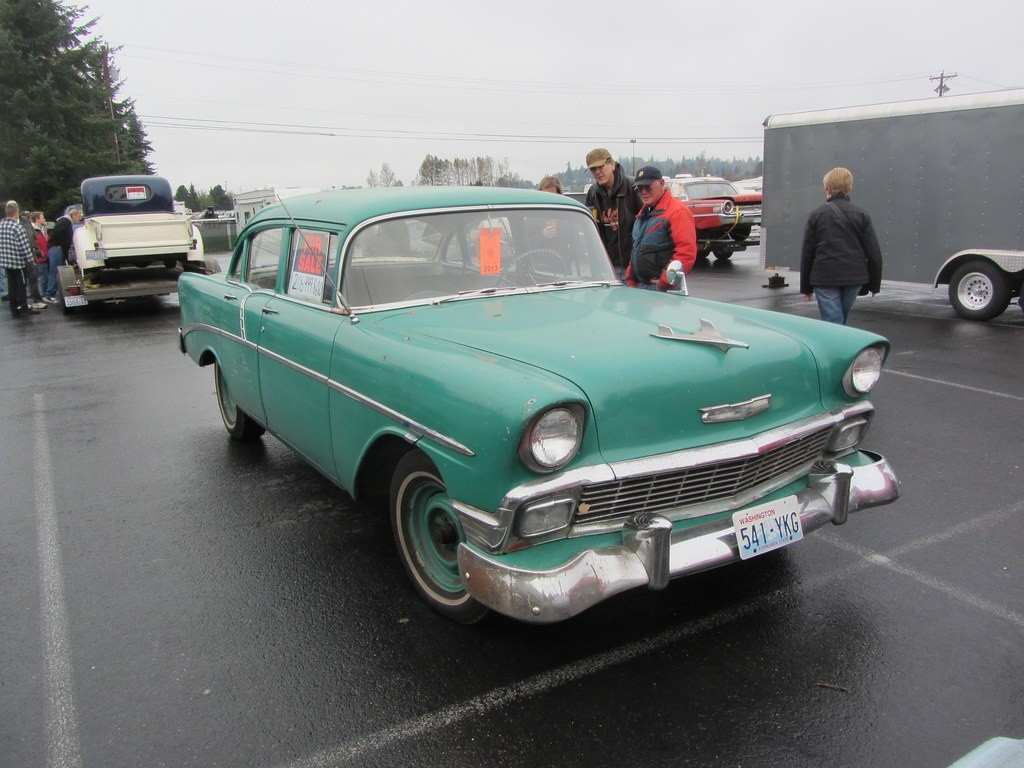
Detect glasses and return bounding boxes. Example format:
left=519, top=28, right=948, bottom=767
left=634, top=183, right=659, bottom=191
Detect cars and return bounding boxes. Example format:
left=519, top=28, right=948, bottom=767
left=64, top=174, right=204, bottom=286
left=176, top=185, right=908, bottom=634
left=223, top=172, right=762, bottom=273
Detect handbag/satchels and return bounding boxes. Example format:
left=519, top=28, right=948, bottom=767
left=858, top=284, right=869, bottom=295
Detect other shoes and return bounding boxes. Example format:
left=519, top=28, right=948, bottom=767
left=32, top=303, right=47, bottom=308
left=42, top=297, right=57, bottom=304
left=17, top=305, right=32, bottom=309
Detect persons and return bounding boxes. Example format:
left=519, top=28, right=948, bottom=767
left=584, top=149, right=644, bottom=286
left=626, top=167, right=697, bottom=292
left=799, top=167, right=882, bottom=326
left=539, top=176, right=563, bottom=195
left=0, top=201, right=83, bottom=320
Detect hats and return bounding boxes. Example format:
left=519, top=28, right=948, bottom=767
left=584, top=148, right=611, bottom=172
left=631, top=166, right=662, bottom=187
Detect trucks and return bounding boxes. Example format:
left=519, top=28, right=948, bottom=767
left=757, top=84, right=1024, bottom=326
